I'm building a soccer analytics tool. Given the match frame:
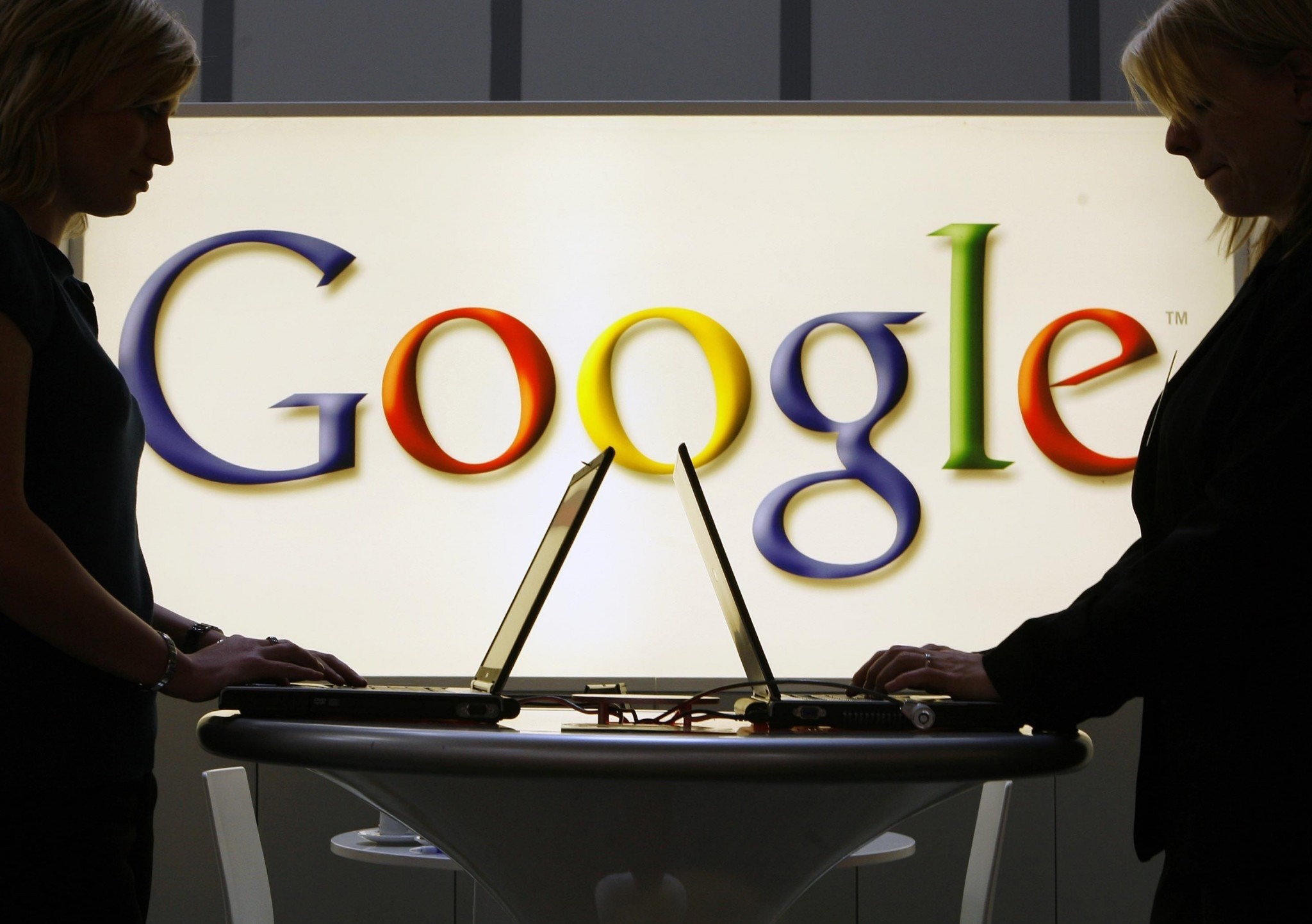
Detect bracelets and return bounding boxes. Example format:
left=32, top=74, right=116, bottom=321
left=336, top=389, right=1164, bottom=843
left=180, top=623, right=221, bottom=656
left=139, top=630, right=178, bottom=691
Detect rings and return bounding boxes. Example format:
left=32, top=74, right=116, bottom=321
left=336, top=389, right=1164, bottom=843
left=924, top=652, right=934, bottom=668
left=266, top=636, right=278, bottom=645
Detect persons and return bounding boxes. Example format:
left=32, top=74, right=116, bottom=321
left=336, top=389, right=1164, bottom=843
left=845, top=1, right=1312, bottom=924
left=0, top=1, right=369, bottom=923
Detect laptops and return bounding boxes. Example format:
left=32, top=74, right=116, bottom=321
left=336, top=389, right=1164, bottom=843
left=672, top=442, right=1023, bottom=731
left=217, top=446, right=616, bottom=723
